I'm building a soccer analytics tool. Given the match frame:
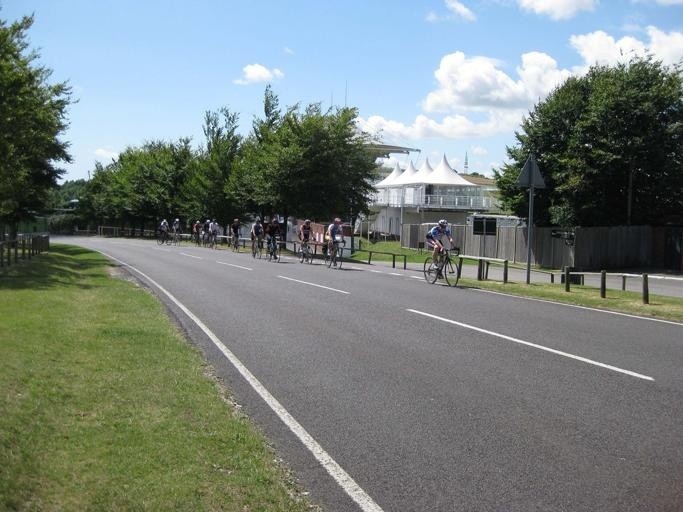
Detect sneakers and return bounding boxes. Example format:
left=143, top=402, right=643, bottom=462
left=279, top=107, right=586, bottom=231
left=431, top=263, right=438, bottom=270
left=438, top=274, right=442, bottom=278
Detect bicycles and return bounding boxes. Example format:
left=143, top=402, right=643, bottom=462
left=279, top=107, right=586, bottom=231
left=325, top=240, right=345, bottom=269
left=423, top=248, right=460, bottom=287
left=252, top=232, right=262, bottom=259
left=266, top=234, right=280, bottom=263
left=193, top=232, right=220, bottom=250
left=157, top=229, right=181, bottom=247
left=298, top=239, right=314, bottom=264
left=230, top=234, right=242, bottom=253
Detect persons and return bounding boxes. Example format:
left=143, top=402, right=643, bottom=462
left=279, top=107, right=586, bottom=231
left=160, top=216, right=282, bottom=259
left=324, top=217, right=345, bottom=266
left=299, top=220, right=315, bottom=252
left=426, top=219, right=453, bottom=279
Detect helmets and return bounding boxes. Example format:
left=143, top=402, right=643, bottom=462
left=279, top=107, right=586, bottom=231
left=439, top=219, right=447, bottom=225
